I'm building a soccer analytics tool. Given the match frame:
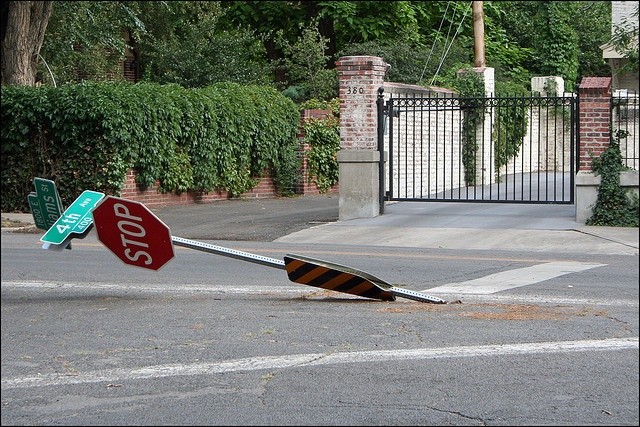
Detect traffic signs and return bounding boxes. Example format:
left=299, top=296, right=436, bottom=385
left=40, top=190, right=105, bottom=246
left=28, top=177, right=72, bottom=250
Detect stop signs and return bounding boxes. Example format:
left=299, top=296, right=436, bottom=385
left=92, top=195, right=175, bottom=271
